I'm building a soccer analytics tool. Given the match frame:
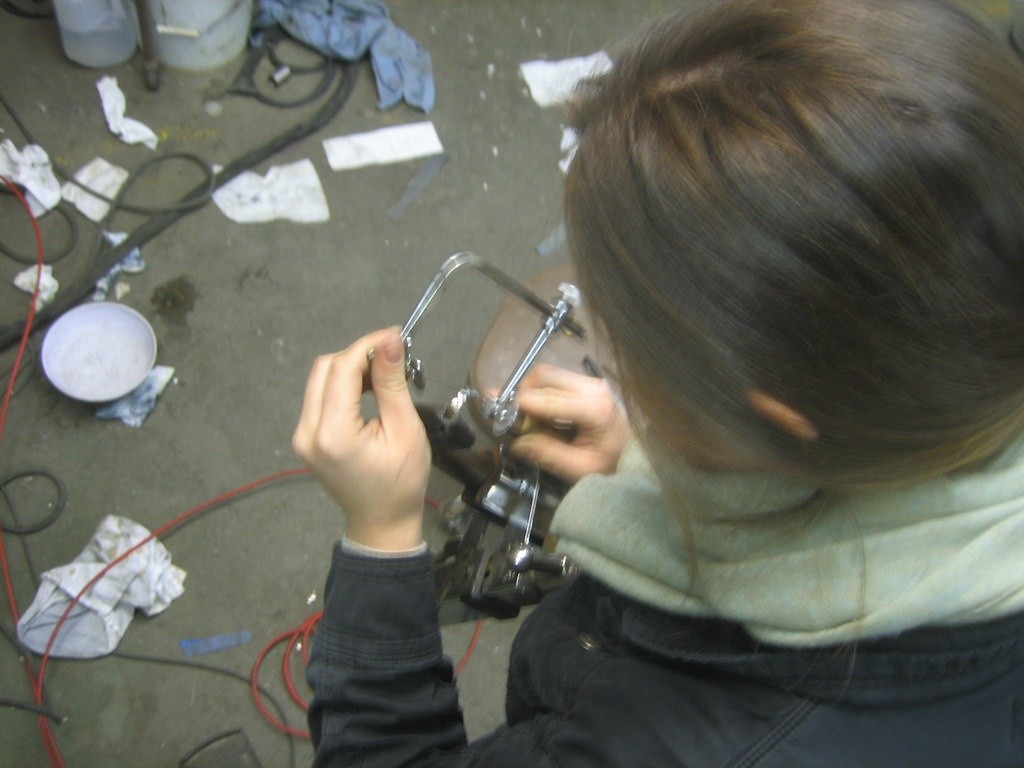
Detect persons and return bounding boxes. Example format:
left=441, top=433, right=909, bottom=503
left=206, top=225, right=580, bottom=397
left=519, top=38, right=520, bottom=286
left=292, top=1, right=1024, bottom=768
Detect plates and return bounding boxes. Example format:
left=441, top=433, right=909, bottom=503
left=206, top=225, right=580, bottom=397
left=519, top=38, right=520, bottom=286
left=43, top=301, right=158, bottom=403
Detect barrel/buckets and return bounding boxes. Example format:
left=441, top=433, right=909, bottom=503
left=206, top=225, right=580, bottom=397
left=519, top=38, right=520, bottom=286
left=141, top=0, right=253, bottom=71
left=54, top=0, right=145, bottom=67
